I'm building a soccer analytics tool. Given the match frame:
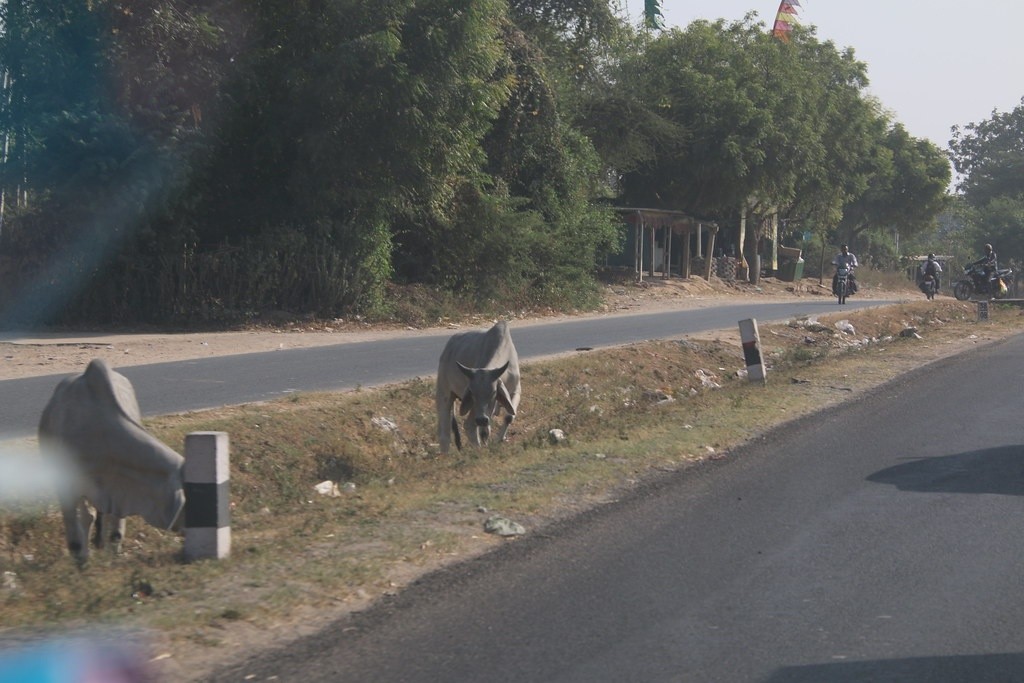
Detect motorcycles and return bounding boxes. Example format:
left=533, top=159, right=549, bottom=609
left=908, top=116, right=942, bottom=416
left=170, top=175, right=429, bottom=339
left=920, top=269, right=942, bottom=301
left=831, top=261, right=858, bottom=305
left=954, top=255, right=1016, bottom=300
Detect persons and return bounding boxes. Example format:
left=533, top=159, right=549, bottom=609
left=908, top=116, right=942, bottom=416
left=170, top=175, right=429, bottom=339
left=831, top=244, right=858, bottom=294
left=919, top=253, right=942, bottom=292
left=972, top=244, right=998, bottom=301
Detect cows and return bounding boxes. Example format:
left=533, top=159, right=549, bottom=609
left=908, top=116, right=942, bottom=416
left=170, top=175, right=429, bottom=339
left=37, top=356, right=189, bottom=572
left=434, top=320, right=522, bottom=459
